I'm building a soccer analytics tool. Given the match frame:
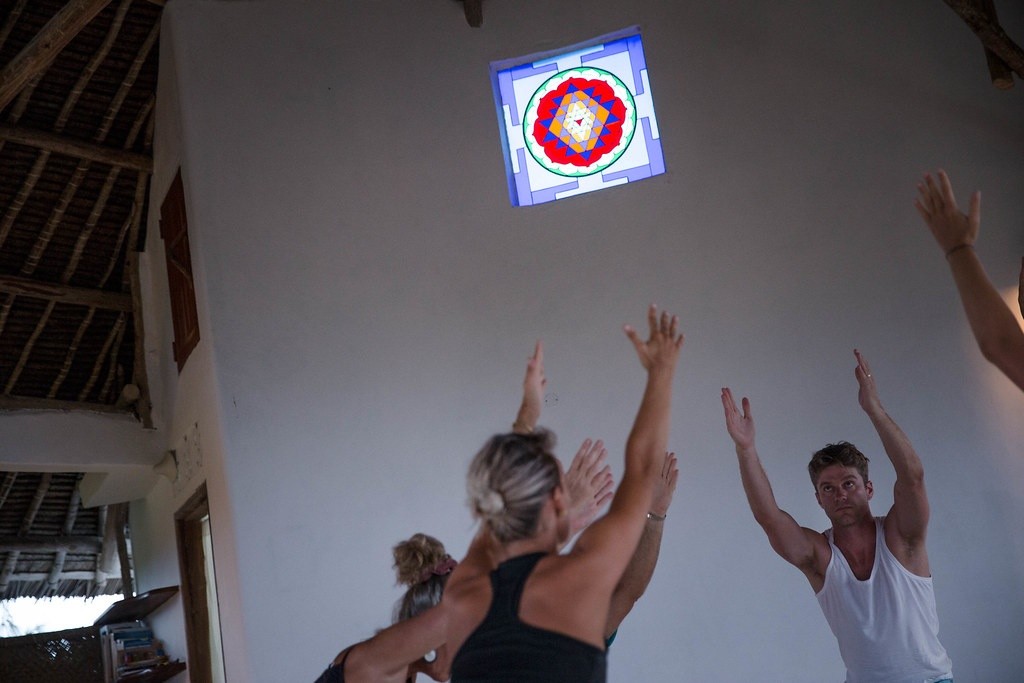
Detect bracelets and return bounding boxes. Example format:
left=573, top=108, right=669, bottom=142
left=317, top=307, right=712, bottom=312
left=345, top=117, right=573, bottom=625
left=945, top=244, right=975, bottom=260
left=647, top=513, right=666, bottom=521
left=512, top=422, right=533, bottom=433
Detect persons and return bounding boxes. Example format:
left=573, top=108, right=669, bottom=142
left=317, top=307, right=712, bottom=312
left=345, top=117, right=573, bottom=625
left=443, top=302, right=685, bottom=683
left=722, top=349, right=953, bottom=683
left=313, top=438, right=679, bottom=683
left=913, top=169, right=1024, bottom=391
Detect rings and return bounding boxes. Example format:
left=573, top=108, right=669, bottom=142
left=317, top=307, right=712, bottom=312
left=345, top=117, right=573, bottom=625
left=868, top=375, right=870, bottom=377
left=663, top=330, right=670, bottom=335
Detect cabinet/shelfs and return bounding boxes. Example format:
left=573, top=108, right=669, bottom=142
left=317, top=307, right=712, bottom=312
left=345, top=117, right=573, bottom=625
left=94, top=585, right=188, bottom=683
